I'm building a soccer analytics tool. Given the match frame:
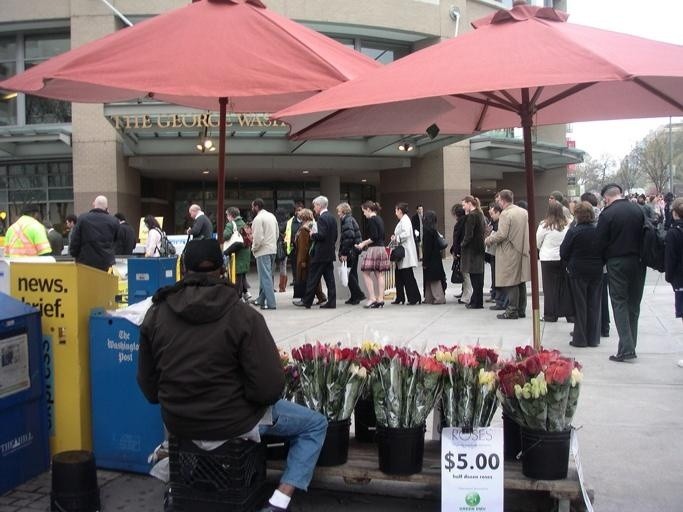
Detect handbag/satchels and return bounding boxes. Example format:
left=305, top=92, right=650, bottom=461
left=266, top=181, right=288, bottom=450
left=436, top=231, right=448, bottom=250
left=451, top=258, right=464, bottom=283
left=389, top=238, right=405, bottom=261
left=338, top=260, right=351, bottom=287
left=222, top=221, right=244, bottom=255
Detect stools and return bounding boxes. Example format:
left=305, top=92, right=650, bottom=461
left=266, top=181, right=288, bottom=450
left=163, top=432, right=268, bottom=512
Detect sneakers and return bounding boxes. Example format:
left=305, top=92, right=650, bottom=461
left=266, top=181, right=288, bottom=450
left=239, top=290, right=527, bottom=321
left=261, top=502, right=289, bottom=512
left=540, top=315, right=612, bottom=348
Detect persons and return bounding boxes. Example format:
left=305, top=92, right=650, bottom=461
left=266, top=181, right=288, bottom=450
left=138, top=238, right=328, bottom=512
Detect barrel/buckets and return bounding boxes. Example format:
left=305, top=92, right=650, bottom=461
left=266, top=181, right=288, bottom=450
left=353, top=395, right=377, bottom=443
left=517, top=426, right=573, bottom=481
left=316, top=414, right=352, bottom=467
left=501, top=405, right=523, bottom=462
left=50, top=449, right=102, bottom=512
left=374, top=420, right=427, bottom=476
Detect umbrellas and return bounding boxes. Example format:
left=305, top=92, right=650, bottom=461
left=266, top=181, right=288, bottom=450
left=264, top=1, right=683, bottom=352
left=0, top=1, right=387, bottom=247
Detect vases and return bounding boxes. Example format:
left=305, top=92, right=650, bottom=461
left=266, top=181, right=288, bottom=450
left=285, top=402, right=571, bottom=479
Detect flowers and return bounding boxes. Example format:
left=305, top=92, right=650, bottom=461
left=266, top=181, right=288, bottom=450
left=276, top=340, right=584, bottom=430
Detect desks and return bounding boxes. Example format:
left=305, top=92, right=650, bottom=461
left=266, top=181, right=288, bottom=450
left=155, top=436, right=595, bottom=512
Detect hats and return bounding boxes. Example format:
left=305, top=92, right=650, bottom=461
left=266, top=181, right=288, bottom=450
left=181, top=236, right=223, bottom=272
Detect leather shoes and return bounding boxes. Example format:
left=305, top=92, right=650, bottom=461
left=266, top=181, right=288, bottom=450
left=609, top=351, right=635, bottom=361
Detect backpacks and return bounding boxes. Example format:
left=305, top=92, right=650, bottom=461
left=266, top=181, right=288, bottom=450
left=153, top=227, right=175, bottom=258
left=239, top=227, right=252, bottom=247
